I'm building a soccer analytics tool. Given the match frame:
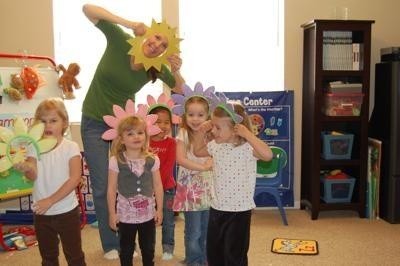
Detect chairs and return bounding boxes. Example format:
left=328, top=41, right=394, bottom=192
left=253, top=152, right=289, bottom=225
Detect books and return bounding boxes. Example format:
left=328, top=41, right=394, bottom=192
left=321, top=28, right=363, bottom=71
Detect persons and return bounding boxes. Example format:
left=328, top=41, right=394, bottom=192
left=193, top=103, right=273, bottom=266
left=146, top=107, right=177, bottom=260
left=81, top=4, right=185, bottom=260
left=175, top=97, right=214, bottom=266
left=106, top=117, right=164, bottom=266
left=12, top=96, right=85, bottom=266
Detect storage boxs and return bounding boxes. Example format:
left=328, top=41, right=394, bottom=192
left=320, top=172, right=356, bottom=204
left=321, top=132, right=354, bottom=160
left=323, top=93, right=364, bottom=116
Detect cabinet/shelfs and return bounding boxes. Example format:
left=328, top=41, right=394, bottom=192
left=300, top=18, right=375, bottom=220
left=374, top=62, right=399, bottom=225
